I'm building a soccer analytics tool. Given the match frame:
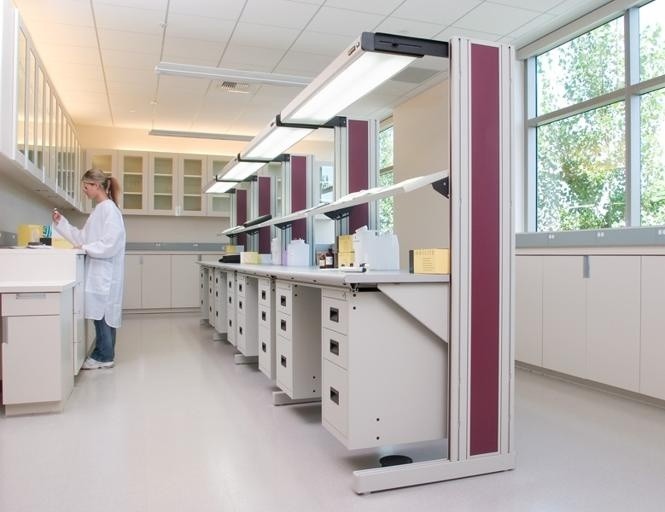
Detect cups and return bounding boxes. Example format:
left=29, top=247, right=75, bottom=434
left=18, top=224, right=53, bottom=250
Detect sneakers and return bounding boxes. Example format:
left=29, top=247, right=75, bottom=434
left=82, top=356, right=114, bottom=369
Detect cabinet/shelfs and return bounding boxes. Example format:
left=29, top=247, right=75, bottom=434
left=0, top=246, right=97, bottom=388
left=0, top=1, right=84, bottom=213
left=84, top=149, right=149, bottom=218
left=149, top=153, right=210, bottom=217
left=0, top=280, right=75, bottom=417
left=172, top=250, right=221, bottom=314
left=123, top=251, right=173, bottom=316
left=193, top=30, right=519, bottom=493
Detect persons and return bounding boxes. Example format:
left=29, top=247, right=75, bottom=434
left=52, top=167, right=126, bottom=370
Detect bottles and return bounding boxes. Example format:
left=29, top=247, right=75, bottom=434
left=326, top=248, right=334, bottom=267
left=319, top=254, right=326, bottom=269
